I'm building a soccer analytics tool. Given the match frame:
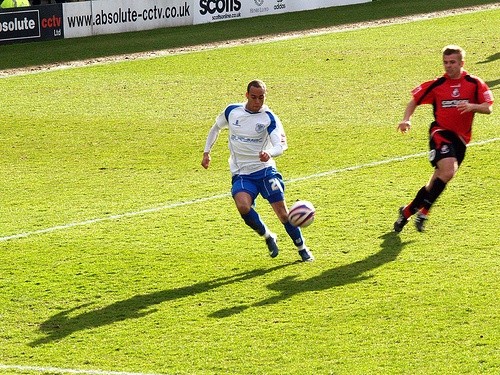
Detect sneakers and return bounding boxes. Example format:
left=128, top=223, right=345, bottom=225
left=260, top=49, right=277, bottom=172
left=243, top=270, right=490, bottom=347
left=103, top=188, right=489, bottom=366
left=394, top=206, right=408, bottom=232
left=266, top=234, right=278, bottom=258
left=416, top=214, right=428, bottom=232
left=298, top=248, right=313, bottom=262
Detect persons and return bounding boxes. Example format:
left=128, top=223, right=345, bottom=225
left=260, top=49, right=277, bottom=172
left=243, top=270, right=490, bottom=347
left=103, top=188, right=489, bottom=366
left=201, top=80, right=314, bottom=261
left=394, top=46, right=494, bottom=233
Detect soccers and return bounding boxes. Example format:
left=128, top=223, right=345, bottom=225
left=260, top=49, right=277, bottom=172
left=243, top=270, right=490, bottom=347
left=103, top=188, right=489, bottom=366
left=288, top=200, right=316, bottom=228
